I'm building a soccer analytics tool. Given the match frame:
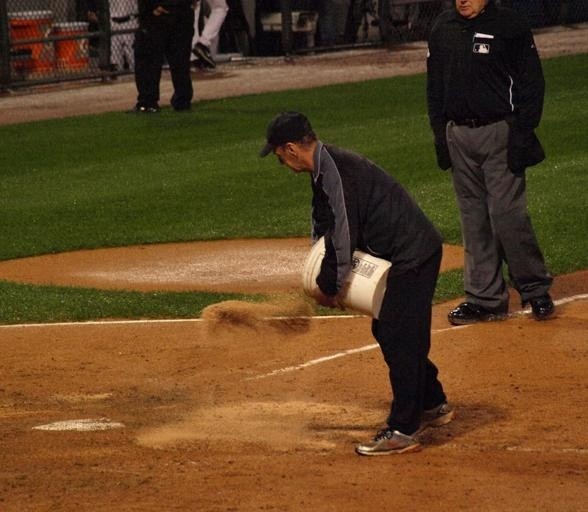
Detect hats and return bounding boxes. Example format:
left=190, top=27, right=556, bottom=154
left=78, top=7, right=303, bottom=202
left=259, top=110, right=311, bottom=158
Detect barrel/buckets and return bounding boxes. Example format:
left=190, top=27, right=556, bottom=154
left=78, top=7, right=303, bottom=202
left=7, top=10, right=56, bottom=78
left=302, top=237, right=393, bottom=320
left=51, top=22, right=90, bottom=74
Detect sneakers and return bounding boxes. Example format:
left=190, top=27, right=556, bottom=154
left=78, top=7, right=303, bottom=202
left=191, top=42, right=220, bottom=72
left=418, top=398, right=454, bottom=435
left=125, top=102, right=159, bottom=112
left=528, top=292, right=555, bottom=321
left=356, top=429, right=422, bottom=455
left=448, top=292, right=510, bottom=324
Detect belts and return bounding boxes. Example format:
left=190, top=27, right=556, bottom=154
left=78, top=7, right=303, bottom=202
left=451, top=115, right=506, bottom=127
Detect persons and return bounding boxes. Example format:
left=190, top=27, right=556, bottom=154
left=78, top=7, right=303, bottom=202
left=188, top=1, right=229, bottom=74
left=89, top=1, right=144, bottom=77
left=220, top=0, right=455, bottom=56
left=258, top=108, right=456, bottom=455
left=124, top=1, right=194, bottom=117
left=425, top=0, right=555, bottom=323
